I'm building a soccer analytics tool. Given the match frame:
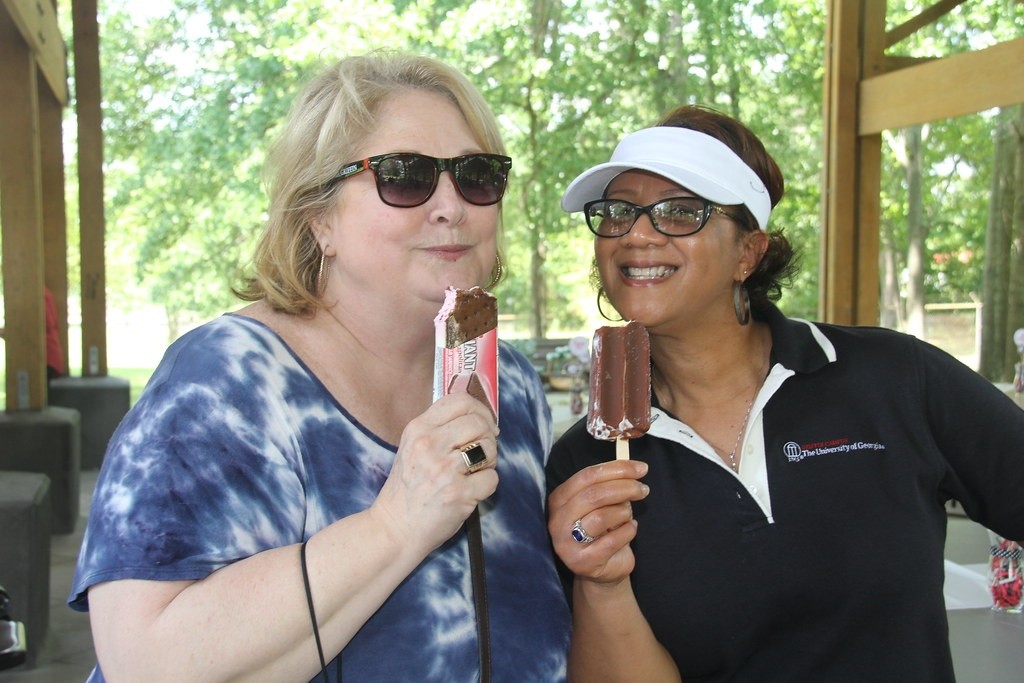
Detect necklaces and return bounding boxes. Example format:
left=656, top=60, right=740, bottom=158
left=708, top=399, right=754, bottom=474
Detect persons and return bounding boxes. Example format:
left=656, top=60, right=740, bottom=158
left=546, top=103, right=1024, bottom=683
left=67, top=47, right=574, bottom=683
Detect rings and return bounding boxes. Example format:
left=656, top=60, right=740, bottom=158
left=459, top=441, right=489, bottom=474
left=570, top=518, right=600, bottom=543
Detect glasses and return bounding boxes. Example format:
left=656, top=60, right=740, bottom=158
left=325, top=152, right=512, bottom=208
left=583, top=196, right=754, bottom=238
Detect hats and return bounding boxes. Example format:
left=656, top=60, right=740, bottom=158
left=562, top=126, right=771, bottom=233
